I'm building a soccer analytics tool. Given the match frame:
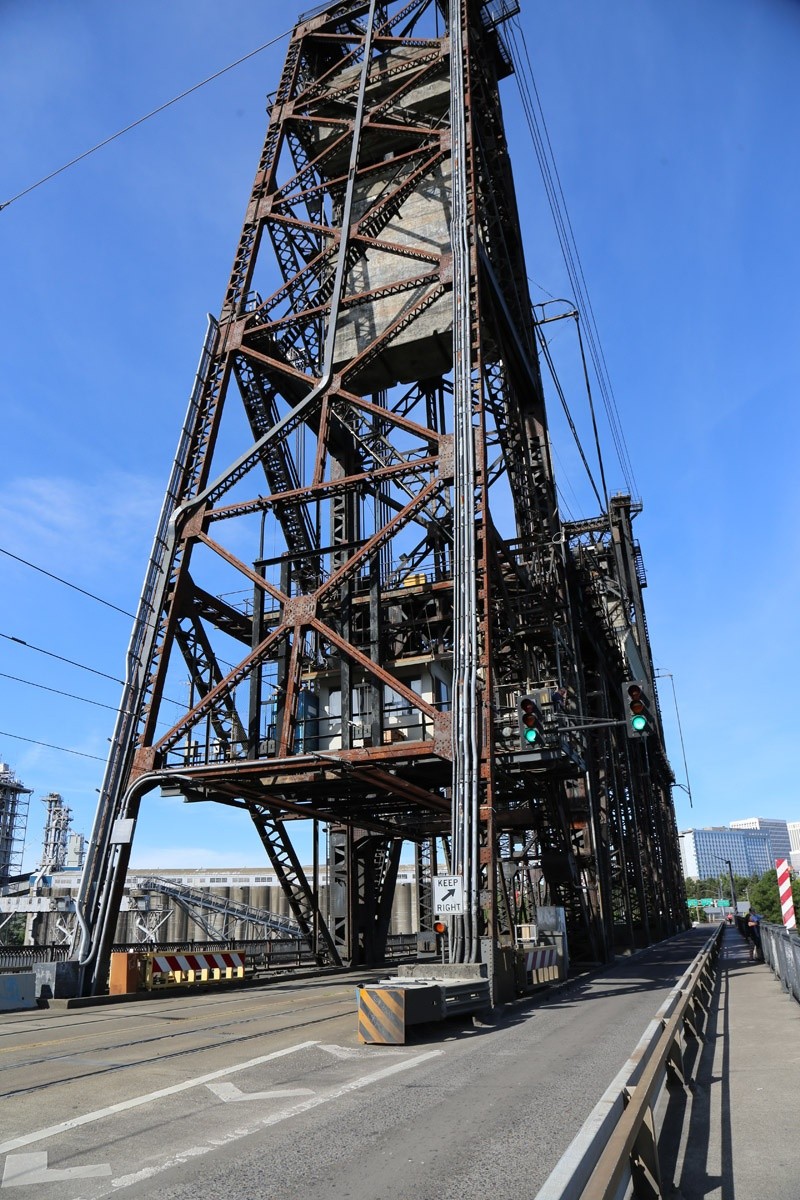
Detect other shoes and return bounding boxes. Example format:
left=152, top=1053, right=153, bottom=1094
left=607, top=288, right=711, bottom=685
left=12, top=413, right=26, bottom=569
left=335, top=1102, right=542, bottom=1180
left=748, top=959, right=757, bottom=963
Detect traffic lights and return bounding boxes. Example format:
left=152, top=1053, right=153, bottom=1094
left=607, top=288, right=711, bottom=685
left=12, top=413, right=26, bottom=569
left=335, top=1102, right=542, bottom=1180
left=621, top=680, right=654, bottom=738
left=432, top=921, right=448, bottom=934
left=516, top=693, right=546, bottom=749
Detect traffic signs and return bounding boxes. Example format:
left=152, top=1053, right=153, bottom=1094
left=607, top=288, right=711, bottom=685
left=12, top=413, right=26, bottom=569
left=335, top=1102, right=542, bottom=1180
left=430, top=875, right=464, bottom=916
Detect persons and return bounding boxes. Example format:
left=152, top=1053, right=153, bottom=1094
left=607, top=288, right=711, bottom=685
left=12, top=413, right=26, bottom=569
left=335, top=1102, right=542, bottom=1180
left=728, top=914, right=732, bottom=924
left=745, top=914, right=759, bottom=961
left=749, top=907, right=765, bottom=960
